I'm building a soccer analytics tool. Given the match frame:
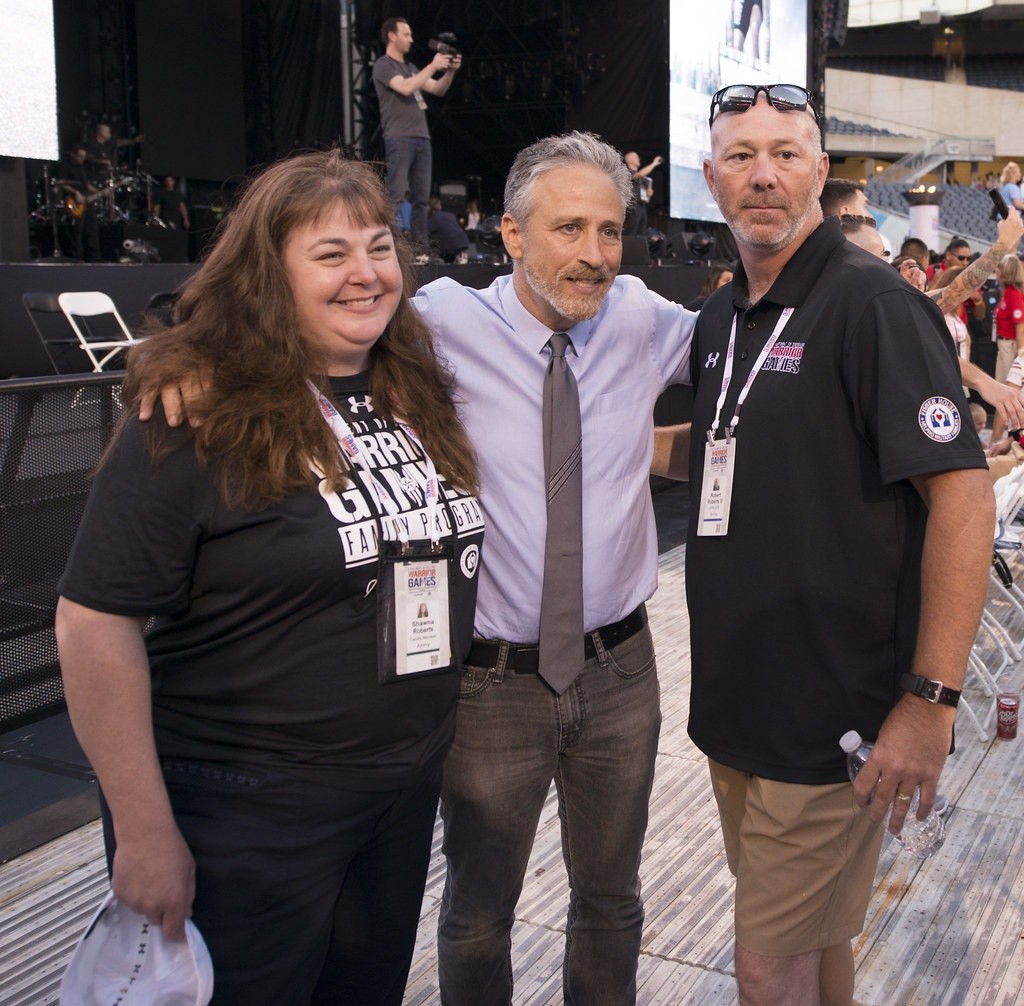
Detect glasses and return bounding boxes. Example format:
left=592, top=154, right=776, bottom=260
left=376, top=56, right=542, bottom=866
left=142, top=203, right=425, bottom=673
left=953, top=254, right=971, bottom=261
left=707, top=84, right=821, bottom=125
left=882, top=250, right=890, bottom=259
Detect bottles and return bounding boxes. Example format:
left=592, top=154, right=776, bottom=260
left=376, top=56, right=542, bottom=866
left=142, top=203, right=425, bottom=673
left=838, top=731, right=944, bottom=858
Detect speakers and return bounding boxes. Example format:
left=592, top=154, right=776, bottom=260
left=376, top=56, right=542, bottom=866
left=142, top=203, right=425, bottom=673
left=622, top=235, right=651, bottom=265
left=658, top=232, right=702, bottom=264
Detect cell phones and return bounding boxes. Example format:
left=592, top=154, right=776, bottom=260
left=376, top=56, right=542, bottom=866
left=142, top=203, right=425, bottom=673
left=988, top=188, right=1009, bottom=220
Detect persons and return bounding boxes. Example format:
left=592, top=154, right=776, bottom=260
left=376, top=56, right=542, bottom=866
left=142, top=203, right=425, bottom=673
left=369, top=15, right=462, bottom=263
left=138, top=130, right=700, bottom=1006
left=55, top=154, right=485, bottom=1005
left=643, top=82, right=996, bottom=1006
left=815, top=157, right=1023, bottom=465
left=71, top=123, right=662, bottom=265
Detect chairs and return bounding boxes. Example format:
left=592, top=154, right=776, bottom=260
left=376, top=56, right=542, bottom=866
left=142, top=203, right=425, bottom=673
left=58, top=290, right=150, bottom=410
left=960, top=461, right=1024, bottom=741
left=110, top=291, right=178, bottom=368
left=20, top=291, right=128, bottom=375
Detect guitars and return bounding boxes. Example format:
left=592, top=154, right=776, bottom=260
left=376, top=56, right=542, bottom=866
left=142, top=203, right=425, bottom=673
left=65, top=176, right=133, bottom=219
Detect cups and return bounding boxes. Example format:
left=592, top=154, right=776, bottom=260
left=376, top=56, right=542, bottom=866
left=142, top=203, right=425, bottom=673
left=996, top=692, right=1018, bottom=740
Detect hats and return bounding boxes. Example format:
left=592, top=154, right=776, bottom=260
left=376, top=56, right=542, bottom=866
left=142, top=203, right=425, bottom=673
left=58, top=888, right=212, bottom=1006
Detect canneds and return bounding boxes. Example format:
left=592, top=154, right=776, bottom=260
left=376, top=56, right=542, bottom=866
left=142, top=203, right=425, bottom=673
left=998, top=698, right=1018, bottom=740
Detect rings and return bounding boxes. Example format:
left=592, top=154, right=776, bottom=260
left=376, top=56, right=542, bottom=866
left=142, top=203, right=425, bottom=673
left=895, top=791, right=910, bottom=801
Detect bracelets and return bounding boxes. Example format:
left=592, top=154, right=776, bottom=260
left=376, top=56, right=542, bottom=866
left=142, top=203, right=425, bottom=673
left=897, top=672, right=962, bottom=708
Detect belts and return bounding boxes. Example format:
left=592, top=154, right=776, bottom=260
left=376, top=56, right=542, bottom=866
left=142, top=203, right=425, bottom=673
left=465, top=601, right=648, bottom=672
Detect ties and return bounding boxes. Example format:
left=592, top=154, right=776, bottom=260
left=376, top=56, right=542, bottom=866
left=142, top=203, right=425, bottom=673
left=540, top=333, right=584, bottom=694
